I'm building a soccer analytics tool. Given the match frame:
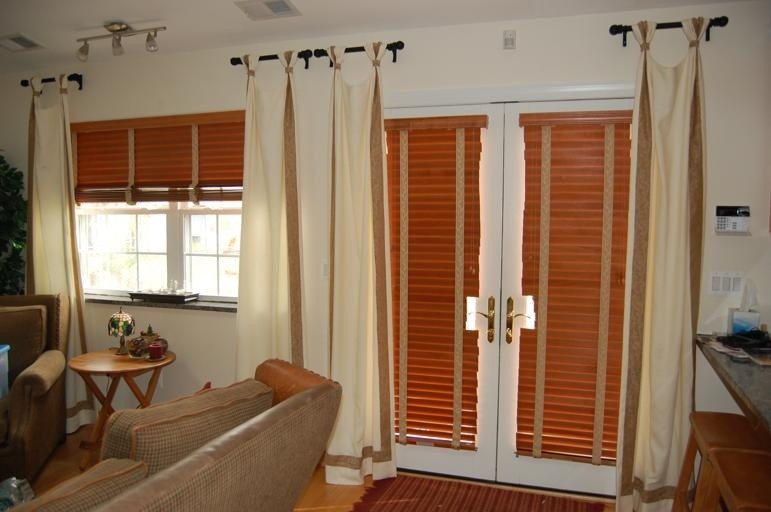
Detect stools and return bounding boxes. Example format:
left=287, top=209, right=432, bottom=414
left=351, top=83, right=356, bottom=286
left=671, top=410, right=766, bottom=512
left=691, top=448, right=771, bottom=512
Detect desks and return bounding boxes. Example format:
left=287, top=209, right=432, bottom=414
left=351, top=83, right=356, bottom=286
left=68, top=350, right=176, bottom=471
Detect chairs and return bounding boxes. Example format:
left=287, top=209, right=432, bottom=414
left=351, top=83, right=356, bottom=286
left=0, top=293, right=70, bottom=482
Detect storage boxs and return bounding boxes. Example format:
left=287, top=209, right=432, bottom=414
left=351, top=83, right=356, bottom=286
left=0, top=344, right=12, bottom=399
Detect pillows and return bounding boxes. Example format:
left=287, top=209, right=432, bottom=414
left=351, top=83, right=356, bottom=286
left=4, top=457, right=149, bottom=512
left=100, top=379, right=274, bottom=476
left=0, top=305, right=47, bottom=386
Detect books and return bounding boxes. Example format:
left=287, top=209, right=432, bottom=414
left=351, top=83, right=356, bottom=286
left=130, top=291, right=200, bottom=303
left=709, top=328, right=771, bottom=366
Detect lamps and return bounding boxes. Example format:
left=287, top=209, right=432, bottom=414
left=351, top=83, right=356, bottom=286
left=76, top=22, right=166, bottom=62
left=107, top=306, right=135, bottom=356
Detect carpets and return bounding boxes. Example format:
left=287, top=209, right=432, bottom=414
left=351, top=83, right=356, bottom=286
left=349, top=474, right=604, bottom=512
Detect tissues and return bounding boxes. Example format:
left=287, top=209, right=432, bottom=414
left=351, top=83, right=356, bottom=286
left=729, top=278, right=759, bottom=336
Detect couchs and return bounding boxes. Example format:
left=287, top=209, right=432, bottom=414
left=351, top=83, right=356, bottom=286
left=5, top=358, right=342, bottom=512
